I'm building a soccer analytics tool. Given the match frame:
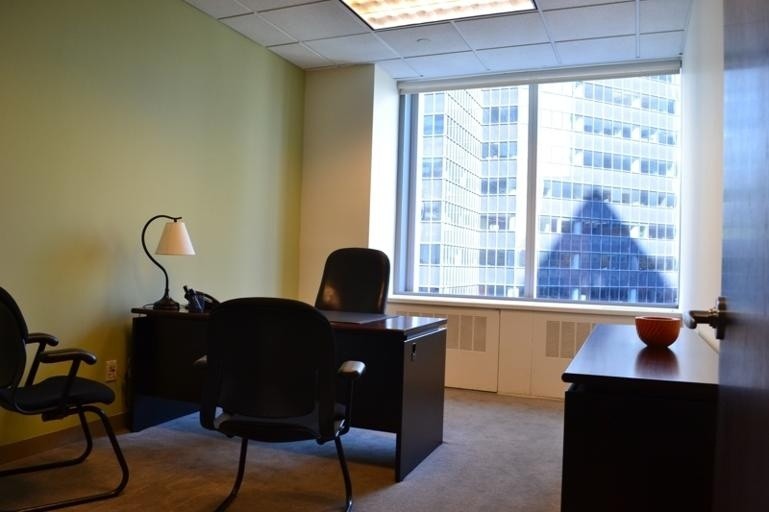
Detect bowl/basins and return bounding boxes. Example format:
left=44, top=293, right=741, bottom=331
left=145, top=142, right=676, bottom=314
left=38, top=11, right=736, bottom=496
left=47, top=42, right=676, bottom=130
left=636, top=316, right=681, bottom=350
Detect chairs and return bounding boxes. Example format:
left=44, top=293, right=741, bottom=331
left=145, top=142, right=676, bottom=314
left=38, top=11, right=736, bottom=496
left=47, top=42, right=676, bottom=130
left=0, top=285, right=129, bottom=512
left=314, top=247, right=390, bottom=314
left=198, top=297, right=366, bottom=511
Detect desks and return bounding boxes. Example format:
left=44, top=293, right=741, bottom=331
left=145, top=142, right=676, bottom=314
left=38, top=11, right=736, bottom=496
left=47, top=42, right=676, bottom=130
left=124, top=308, right=449, bottom=483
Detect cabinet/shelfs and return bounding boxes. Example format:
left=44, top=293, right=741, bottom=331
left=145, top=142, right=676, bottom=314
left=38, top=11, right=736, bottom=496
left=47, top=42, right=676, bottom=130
left=561, top=323, right=720, bottom=511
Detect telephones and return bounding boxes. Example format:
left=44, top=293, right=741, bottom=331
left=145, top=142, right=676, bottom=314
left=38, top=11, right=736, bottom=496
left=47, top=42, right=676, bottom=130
left=183, top=285, right=220, bottom=313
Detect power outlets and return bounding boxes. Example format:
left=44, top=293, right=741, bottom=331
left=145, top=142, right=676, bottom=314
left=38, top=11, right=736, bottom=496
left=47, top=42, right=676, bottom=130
left=105, top=360, right=118, bottom=382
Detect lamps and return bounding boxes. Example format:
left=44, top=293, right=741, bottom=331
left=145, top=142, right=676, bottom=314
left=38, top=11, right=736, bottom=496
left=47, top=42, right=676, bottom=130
left=140, top=215, right=195, bottom=311
